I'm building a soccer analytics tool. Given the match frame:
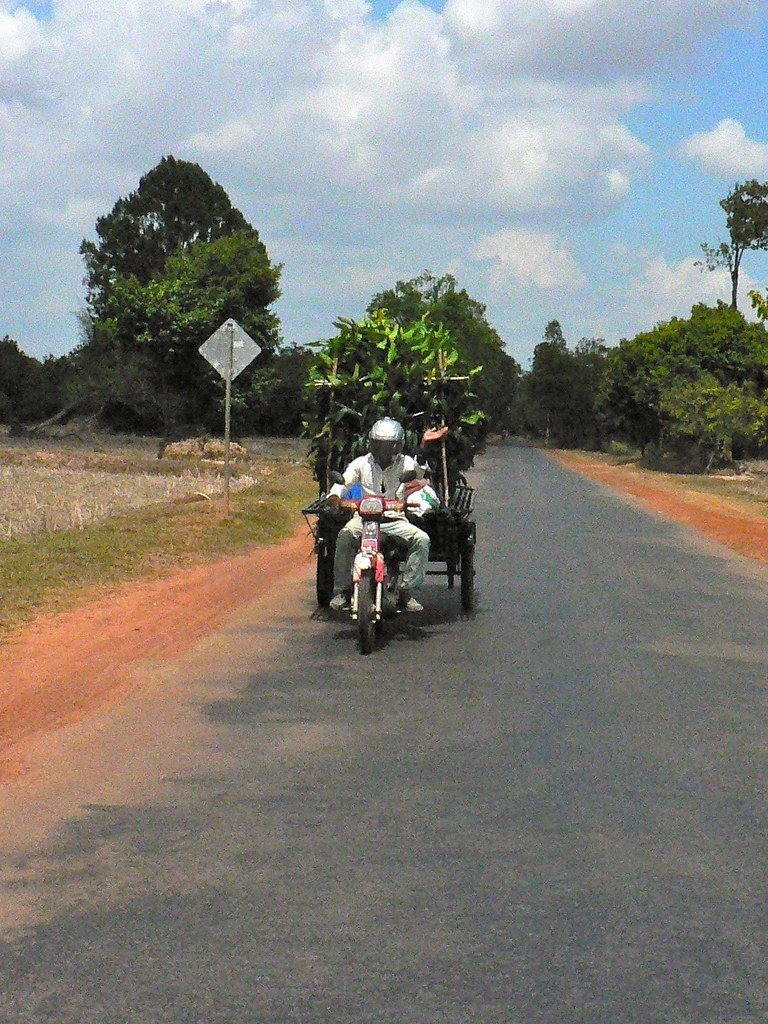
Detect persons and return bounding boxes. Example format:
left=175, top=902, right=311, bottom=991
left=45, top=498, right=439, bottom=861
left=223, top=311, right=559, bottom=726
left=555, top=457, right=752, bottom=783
left=324, top=419, right=448, bottom=612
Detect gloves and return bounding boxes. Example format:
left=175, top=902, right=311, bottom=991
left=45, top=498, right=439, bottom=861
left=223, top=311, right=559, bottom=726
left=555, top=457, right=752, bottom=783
left=321, top=496, right=343, bottom=515
left=403, top=480, right=425, bottom=497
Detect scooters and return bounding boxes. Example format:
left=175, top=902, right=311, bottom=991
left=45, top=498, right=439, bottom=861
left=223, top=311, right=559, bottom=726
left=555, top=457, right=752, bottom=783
left=299, top=480, right=478, bottom=657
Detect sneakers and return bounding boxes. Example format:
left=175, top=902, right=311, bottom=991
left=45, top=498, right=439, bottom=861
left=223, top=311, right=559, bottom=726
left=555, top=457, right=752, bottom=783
left=401, top=596, right=424, bottom=612
left=330, top=594, right=349, bottom=610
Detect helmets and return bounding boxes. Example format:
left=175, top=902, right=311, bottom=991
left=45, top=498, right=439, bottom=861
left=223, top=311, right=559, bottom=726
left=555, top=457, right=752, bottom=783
left=368, top=416, right=406, bottom=464
